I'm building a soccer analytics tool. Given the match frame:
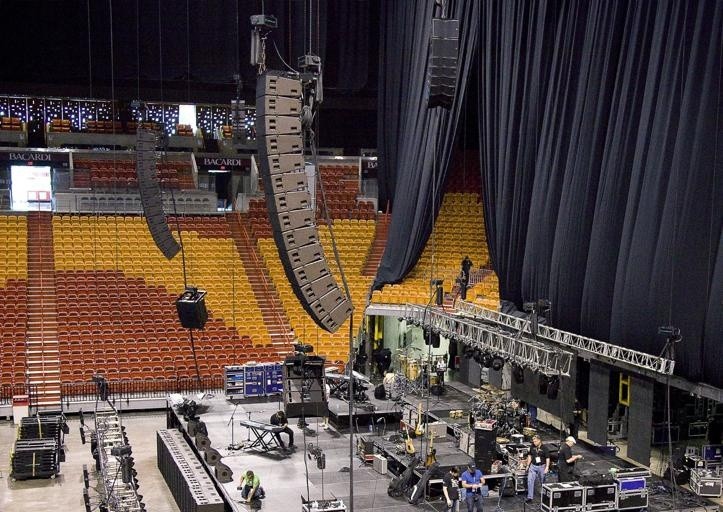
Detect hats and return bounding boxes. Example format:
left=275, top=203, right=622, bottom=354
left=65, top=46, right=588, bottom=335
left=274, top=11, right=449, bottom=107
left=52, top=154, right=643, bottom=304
left=469, top=462, right=476, bottom=468
left=566, top=436, right=577, bottom=445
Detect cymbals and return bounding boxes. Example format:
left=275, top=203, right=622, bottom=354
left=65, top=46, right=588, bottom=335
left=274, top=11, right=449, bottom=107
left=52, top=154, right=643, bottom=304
left=499, top=388, right=510, bottom=391
left=489, top=390, right=504, bottom=395
left=410, top=346, right=422, bottom=351
left=481, top=384, right=497, bottom=391
left=471, top=388, right=486, bottom=394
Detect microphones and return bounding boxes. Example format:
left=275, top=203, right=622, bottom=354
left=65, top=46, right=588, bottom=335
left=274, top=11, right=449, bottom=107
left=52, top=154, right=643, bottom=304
left=235, top=400, right=240, bottom=406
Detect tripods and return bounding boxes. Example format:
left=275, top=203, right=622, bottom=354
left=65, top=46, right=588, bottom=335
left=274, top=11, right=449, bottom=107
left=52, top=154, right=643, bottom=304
left=225, top=404, right=239, bottom=454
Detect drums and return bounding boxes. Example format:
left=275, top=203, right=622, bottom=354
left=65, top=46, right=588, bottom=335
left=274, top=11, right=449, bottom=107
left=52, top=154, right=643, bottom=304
left=510, top=434, right=525, bottom=444
left=467, top=410, right=486, bottom=431
left=522, top=427, right=538, bottom=437
left=429, top=373, right=440, bottom=387
left=406, top=364, right=422, bottom=382
left=506, top=399, right=521, bottom=411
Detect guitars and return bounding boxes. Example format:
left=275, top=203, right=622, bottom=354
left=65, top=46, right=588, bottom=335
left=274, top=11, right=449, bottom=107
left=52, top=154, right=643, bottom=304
left=404, top=421, right=416, bottom=454
left=424, top=429, right=435, bottom=466
left=415, top=403, right=424, bottom=435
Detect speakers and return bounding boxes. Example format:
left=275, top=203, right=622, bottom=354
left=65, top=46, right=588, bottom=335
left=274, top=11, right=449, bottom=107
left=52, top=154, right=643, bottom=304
left=474, top=427, right=499, bottom=470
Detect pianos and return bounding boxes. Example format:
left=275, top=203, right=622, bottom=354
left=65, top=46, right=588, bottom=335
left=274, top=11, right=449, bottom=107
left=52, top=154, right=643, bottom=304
left=240, top=420, right=283, bottom=433
left=325, top=366, right=370, bottom=384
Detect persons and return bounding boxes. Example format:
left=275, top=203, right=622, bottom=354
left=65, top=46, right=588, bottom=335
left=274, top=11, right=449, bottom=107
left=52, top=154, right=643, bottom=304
left=526, top=434, right=550, bottom=503
left=437, top=357, right=446, bottom=386
left=443, top=465, right=460, bottom=512
left=270, top=411, right=298, bottom=449
left=462, top=255, right=473, bottom=281
left=239, top=471, right=264, bottom=503
left=461, top=462, right=485, bottom=512
left=557, top=434, right=583, bottom=506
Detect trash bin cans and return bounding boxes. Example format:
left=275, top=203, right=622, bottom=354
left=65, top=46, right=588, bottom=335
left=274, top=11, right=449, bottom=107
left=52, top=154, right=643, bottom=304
left=11, top=395, right=30, bottom=424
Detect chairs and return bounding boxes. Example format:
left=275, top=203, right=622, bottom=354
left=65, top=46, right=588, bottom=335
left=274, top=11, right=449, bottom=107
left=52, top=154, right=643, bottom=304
left=0, top=117, right=501, bottom=402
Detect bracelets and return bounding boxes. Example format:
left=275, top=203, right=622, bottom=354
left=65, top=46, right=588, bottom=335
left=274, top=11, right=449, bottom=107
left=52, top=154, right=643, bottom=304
left=527, top=464, right=530, bottom=466
left=545, top=465, right=550, bottom=466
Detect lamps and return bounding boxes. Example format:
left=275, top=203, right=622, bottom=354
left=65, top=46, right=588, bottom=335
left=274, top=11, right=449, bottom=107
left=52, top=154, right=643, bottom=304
left=658, top=327, right=682, bottom=343
left=420, top=329, right=559, bottom=400
left=186, top=422, right=235, bottom=484
left=112, top=446, right=134, bottom=482
